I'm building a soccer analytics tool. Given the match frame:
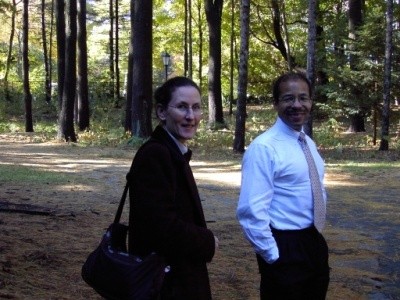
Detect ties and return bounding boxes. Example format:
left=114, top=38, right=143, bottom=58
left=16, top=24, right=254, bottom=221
left=297, top=132, right=325, bottom=233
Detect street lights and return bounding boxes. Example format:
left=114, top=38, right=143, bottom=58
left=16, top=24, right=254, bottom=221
left=161, top=50, right=171, bottom=82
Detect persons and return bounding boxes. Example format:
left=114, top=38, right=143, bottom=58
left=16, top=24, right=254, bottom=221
left=236, top=73, right=330, bottom=300
left=126, top=76, right=219, bottom=300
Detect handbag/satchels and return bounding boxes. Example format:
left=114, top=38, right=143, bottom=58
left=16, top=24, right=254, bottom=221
left=80, top=183, right=170, bottom=300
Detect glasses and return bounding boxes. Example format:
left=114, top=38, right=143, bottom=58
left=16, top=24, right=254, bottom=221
left=281, top=96, right=312, bottom=105
left=167, top=104, right=203, bottom=113
